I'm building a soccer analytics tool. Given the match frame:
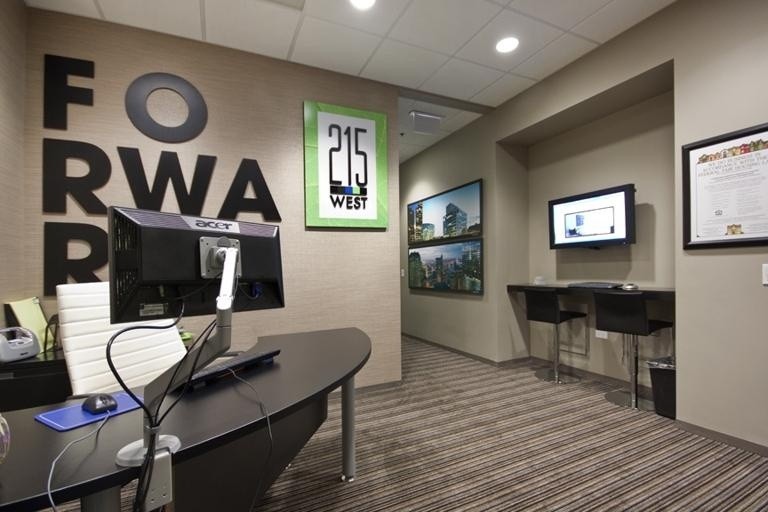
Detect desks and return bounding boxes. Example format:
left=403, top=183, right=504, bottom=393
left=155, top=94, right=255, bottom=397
left=507, top=282, right=675, bottom=302
left=0, top=326, right=372, bottom=512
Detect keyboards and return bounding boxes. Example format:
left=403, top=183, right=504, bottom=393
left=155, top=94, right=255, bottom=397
left=567, top=280, right=622, bottom=290
left=182, top=348, right=282, bottom=389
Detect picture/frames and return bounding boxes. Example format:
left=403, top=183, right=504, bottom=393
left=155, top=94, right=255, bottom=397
left=407, top=178, right=483, bottom=246
left=407, top=238, right=484, bottom=296
left=681, top=121, right=768, bottom=251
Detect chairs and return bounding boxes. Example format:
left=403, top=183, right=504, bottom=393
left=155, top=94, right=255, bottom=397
left=592, top=292, right=672, bottom=411
left=523, top=284, right=587, bottom=384
left=2, top=295, right=56, bottom=354
left=56, top=279, right=189, bottom=512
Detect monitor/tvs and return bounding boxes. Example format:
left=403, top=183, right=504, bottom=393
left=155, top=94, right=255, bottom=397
left=108, top=205, right=287, bottom=325
left=547, top=184, right=636, bottom=248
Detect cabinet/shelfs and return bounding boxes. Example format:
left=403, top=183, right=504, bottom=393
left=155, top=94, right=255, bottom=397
left=0, top=324, right=193, bottom=412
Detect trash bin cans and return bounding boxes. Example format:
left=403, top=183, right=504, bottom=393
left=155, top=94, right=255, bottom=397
left=645, top=355, right=675, bottom=420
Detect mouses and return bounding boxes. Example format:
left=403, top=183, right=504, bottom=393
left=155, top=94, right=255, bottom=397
left=81, top=393, right=116, bottom=413
left=623, top=283, right=639, bottom=291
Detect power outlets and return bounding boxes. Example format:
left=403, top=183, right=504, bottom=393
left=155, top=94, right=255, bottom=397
left=594, top=330, right=609, bottom=341
